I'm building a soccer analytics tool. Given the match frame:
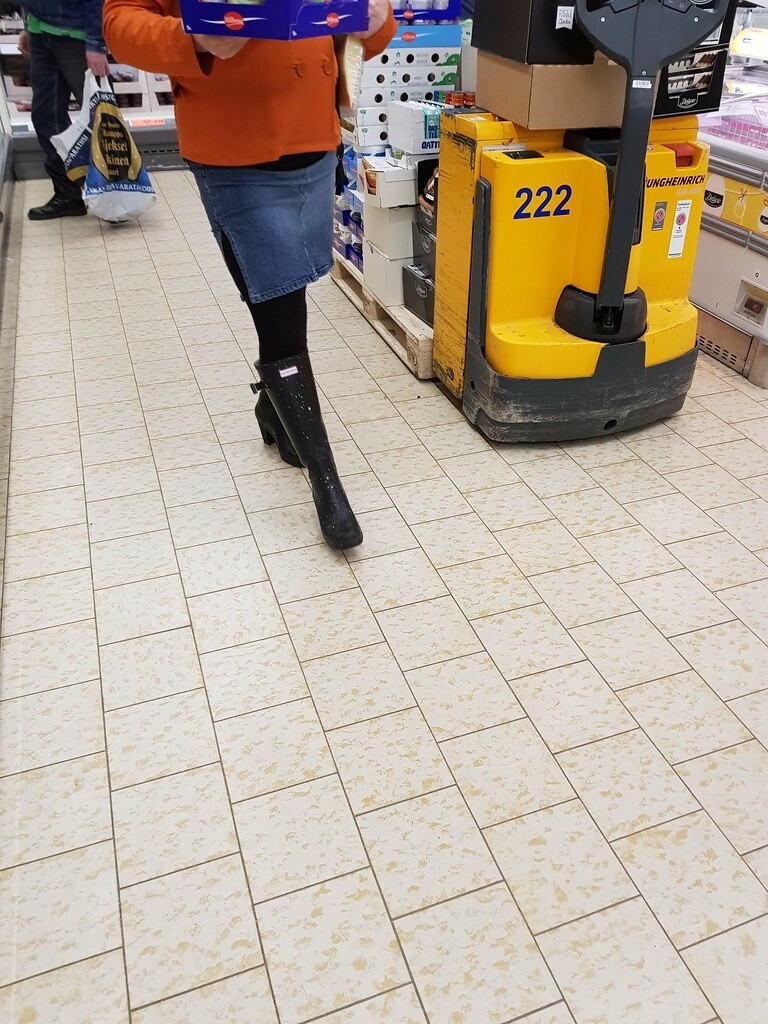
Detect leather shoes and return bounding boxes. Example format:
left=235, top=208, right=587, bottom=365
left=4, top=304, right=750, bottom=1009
left=28, top=193, right=86, bottom=219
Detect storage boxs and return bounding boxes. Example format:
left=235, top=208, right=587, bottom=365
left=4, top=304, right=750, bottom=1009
left=334, top=13, right=729, bottom=327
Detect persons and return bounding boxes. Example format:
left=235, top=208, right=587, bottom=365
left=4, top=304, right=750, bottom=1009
left=16, top=0, right=110, bottom=220
left=101, top=1, right=398, bottom=549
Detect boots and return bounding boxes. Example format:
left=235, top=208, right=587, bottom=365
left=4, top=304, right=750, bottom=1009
left=254, top=390, right=304, bottom=467
left=251, top=348, right=363, bottom=549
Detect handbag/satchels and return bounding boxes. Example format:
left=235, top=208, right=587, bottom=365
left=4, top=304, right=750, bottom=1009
left=49, top=69, right=156, bottom=222
